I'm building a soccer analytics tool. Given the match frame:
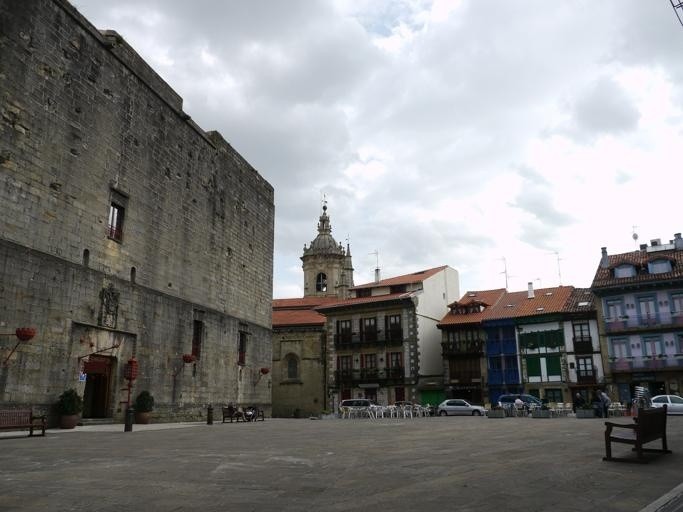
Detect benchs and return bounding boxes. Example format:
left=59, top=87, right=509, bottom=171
left=602, top=404, right=673, bottom=464
left=222, top=404, right=265, bottom=423
left=0, top=407, right=48, bottom=437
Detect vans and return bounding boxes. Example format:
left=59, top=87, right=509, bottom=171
left=338, top=397, right=388, bottom=419
left=499, top=394, right=544, bottom=410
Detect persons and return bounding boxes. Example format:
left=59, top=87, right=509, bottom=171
left=573, top=393, right=585, bottom=413
left=228, top=401, right=257, bottom=422
left=515, top=396, right=525, bottom=410
left=596, top=390, right=611, bottom=418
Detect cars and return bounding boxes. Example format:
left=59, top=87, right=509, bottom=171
left=650, top=394, right=683, bottom=416
left=437, top=397, right=485, bottom=417
left=387, top=400, right=434, bottom=416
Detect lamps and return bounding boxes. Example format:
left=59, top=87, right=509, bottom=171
left=1, top=327, right=36, bottom=363
left=125, top=356, right=138, bottom=380
left=254, top=368, right=269, bottom=387
left=171, top=354, right=197, bottom=377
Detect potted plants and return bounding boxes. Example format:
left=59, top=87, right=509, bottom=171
left=575, top=396, right=596, bottom=418
left=51, top=388, right=85, bottom=429
left=134, top=390, right=155, bottom=423
left=488, top=407, right=506, bottom=418
left=532, top=405, right=552, bottom=418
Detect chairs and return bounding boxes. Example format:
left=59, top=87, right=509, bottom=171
left=341, top=404, right=431, bottom=420
